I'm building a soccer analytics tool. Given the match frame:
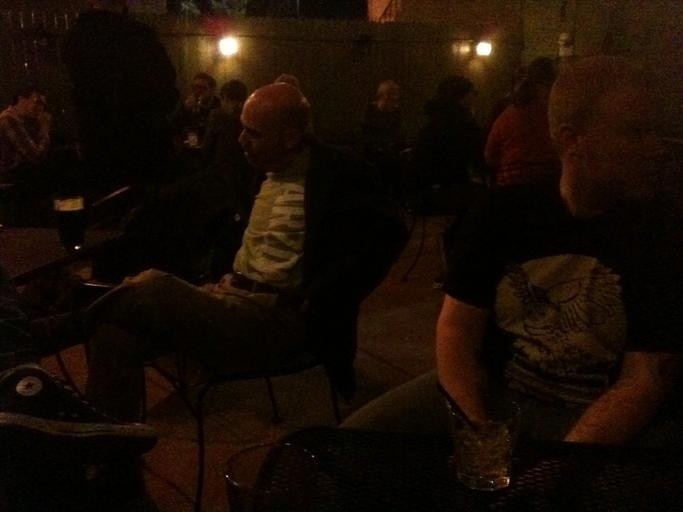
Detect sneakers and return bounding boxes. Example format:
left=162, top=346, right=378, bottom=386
left=28, top=311, right=80, bottom=346
left=0, top=364, right=157, bottom=457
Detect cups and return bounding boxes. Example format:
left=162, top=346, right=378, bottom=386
left=446, top=394, right=522, bottom=491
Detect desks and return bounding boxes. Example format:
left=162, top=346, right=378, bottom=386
left=0, top=226, right=127, bottom=303
left=240, top=424, right=683, bottom=512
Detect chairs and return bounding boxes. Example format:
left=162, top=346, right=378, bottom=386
left=167, top=180, right=368, bottom=509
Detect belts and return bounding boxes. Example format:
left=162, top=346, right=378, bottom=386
left=231, top=271, right=278, bottom=293
